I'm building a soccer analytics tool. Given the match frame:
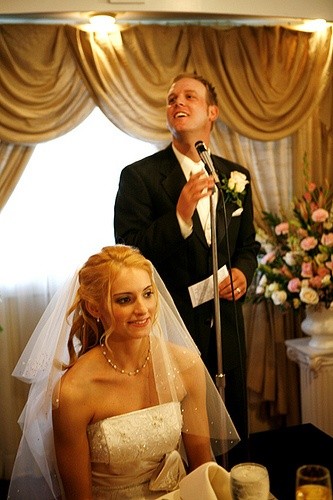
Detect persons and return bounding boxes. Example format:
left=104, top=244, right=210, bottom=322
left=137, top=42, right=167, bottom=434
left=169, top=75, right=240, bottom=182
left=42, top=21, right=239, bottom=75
left=7, top=242, right=240, bottom=500
left=113, top=73, right=262, bottom=472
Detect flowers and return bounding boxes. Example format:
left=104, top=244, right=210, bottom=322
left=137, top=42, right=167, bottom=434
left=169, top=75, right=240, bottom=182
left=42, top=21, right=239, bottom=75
left=246, top=182, right=333, bottom=312
left=222, top=170, right=249, bottom=217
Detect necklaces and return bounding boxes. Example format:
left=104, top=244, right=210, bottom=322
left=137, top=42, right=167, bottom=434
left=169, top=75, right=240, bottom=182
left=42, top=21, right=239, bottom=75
left=101, top=343, right=150, bottom=375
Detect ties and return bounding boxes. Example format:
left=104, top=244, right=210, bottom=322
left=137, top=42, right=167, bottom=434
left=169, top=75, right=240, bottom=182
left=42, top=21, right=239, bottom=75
left=191, top=162, right=212, bottom=247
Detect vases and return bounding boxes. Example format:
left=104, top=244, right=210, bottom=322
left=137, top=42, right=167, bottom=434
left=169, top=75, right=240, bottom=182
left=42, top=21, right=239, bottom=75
left=301, top=302, right=333, bottom=348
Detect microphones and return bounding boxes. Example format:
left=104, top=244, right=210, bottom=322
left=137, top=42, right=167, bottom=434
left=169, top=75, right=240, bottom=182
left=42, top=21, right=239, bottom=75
left=195, top=141, right=223, bottom=187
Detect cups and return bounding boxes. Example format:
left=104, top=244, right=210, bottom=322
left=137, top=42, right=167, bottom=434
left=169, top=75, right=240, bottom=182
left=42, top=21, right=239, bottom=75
left=230, top=463, right=269, bottom=500
left=296, top=465, right=333, bottom=500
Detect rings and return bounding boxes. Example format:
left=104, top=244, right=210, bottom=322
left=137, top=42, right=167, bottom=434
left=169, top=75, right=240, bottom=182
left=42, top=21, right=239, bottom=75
left=236, top=288, right=240, bottom=294
